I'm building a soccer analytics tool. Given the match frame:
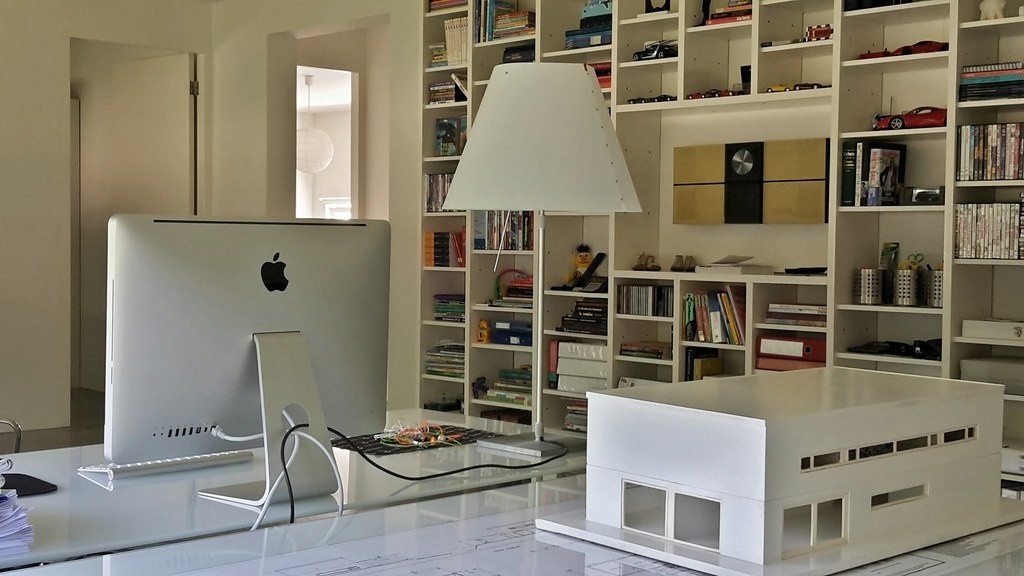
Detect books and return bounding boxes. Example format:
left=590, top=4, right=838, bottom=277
left=955, top=121, right=1024, bottom=180
left=841, top=139, right=907, bottom=208
left=425, top=225, right=467, bottom=268
left=958, top=61, right=1024, bottom=102
left=433, top=294, right=465, bottom=323
left=766, top=303, right=828, bottom=328
left=705, top=0, right=752, bottom=26
left=423, top=0, right=468, bottom=214
left=474, top=210, right=535, bottom=252
left=617, top=284, right=675, bottom=390
left=549, top=298, right=609, bottom=433
left=953, top=202, right=1024, bottom=261
left=486, top=366, right=534, bottom=407
left=681, top=284, right=745, bottom=382
left=426, top=340, right=466, bottom=378
left=492, top=273, right=533, bottom=309
left=472, top=0, right=611, bottom=89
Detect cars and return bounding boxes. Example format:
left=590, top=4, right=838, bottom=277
left=793, top=83, right=822, bottom=90
left=686, top=89, right=737, bottom=99
left=632, top=44, right=678, bottom=61
left=627, top=94, right=677, bottom=104
left=763, top=83, right=794, bottom=93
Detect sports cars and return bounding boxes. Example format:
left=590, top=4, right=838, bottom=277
left=892, top=41, right=949, bottom=55
left=872, top=107, right=946, bottom=130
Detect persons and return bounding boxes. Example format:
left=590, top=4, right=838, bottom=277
left=880, top=156, right=898, bottom=203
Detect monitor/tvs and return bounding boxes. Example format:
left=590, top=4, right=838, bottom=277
left=104, top=213, right=391, bottom=506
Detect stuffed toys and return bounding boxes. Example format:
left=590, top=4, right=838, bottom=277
left=565, top=244, right=599, bottom=285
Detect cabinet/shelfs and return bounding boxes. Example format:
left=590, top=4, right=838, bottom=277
left=416, top=1, right=1024, bottom=498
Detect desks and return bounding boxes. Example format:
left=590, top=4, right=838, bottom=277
left=2, top=406, right=1022, bottom=575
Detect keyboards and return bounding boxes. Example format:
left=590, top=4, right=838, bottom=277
left=78, top=452, right=252, bottom=480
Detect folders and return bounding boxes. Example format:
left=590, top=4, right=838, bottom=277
left=756, top=335, right=826, bottom=363
left=491, top=332, right=533, bottom=347
left=489, top=318, right=533, bottom=334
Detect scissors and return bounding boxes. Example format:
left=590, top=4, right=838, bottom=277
left=900, top=260, right=920, bottom=270
left=908, top=254, right=924, bottom=270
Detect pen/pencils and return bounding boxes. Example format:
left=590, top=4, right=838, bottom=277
left=926, top=264, right=933, bottom=271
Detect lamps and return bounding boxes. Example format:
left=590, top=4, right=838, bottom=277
left=293, top=74, right=334, bottom=173
left=445, top=61, right=641, bottom=458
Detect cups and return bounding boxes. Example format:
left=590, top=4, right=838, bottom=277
left=866, top=186, right=882, bottom=206
